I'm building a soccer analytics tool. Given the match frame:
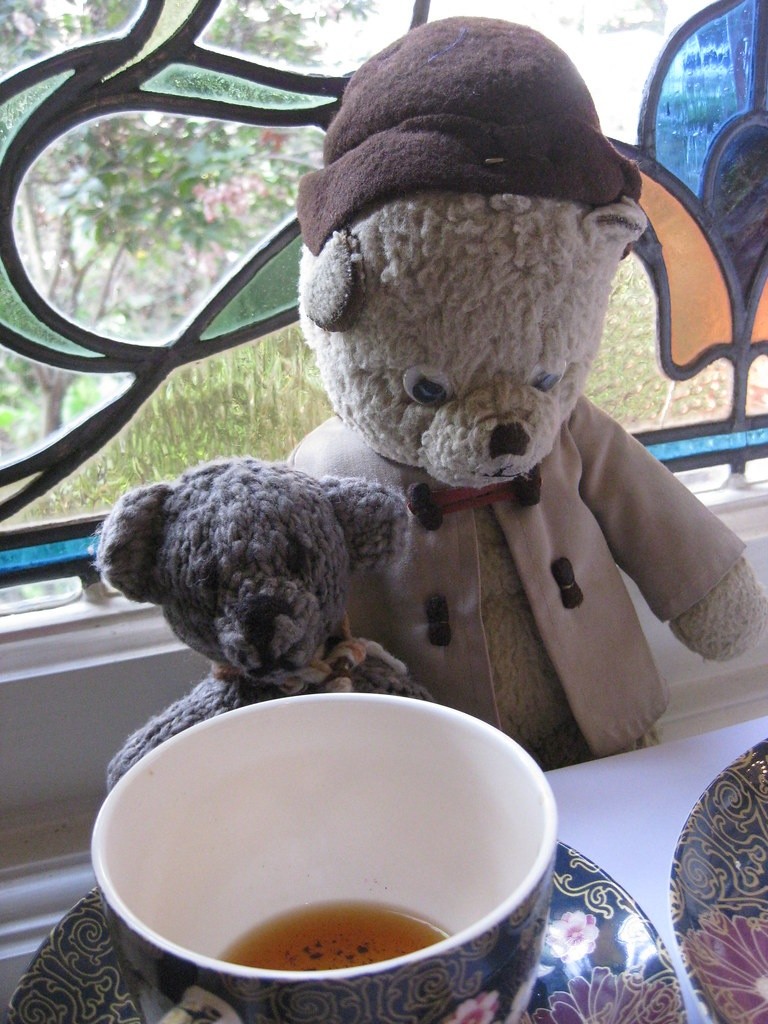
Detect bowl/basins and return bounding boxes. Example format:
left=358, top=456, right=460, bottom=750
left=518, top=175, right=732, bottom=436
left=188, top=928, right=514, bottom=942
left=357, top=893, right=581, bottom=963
left=88, top=693, right=557, bottom=1024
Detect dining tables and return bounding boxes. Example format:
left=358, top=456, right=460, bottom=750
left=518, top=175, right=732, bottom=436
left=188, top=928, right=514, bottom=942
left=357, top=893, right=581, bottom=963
left=0, top=713, right=768, bottom=1024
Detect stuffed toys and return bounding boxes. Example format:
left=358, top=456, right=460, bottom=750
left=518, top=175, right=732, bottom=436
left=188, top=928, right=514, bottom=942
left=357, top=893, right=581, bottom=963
left=94, top=458, right=441, bottom=800
left=274, top=19, right=766, bottom=776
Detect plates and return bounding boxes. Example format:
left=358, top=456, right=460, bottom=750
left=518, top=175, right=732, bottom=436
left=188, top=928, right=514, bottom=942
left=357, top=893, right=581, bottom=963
left=670, top=737, right=767, bottom=1023
left=5, top=838, right=683, bottom=1023
